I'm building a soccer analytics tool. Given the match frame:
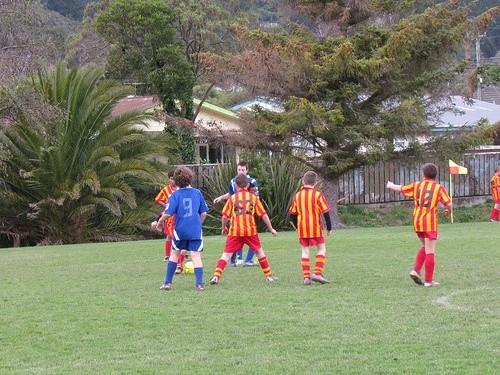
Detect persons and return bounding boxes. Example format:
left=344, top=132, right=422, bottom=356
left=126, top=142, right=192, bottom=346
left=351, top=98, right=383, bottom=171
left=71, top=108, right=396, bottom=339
left=211, top=160, right=262, bottom=267
left=386, top=162, right=452, bottom=288
left=289, top=170, right=333, bottom=286
left=152, top=170, right=188, bottom=275
left=155, top=163, right=208, bottom=292
left=489, top=166, right=500, bottom=222
left=208, top=172, right=278, bottom=285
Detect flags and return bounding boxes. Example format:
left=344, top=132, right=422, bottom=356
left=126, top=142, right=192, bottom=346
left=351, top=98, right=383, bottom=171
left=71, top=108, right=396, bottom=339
left=448, top=159, right=468, bottom=174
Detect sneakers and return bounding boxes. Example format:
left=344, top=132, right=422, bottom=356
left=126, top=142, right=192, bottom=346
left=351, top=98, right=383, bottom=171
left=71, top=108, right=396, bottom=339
left=160, top=284, right=172, bottom=290
left=209, top=277, right=219, bottom=285
left=410, top=269, right=422, bottom=287
left=423, top=280, right=439, bottom=288
left=185, top=254, right=190, bottom=260
left=231, top=262, right=236, bottom=267
left=237, top=259, right=243, bottom=264
left=311, top=273, right=330, bottom=283
left=163, top=257, right=169, bottom=261
left=244, top=260, right=260, bottom=266
left=174, top=266, right=182, bottom=275
left=303, top=279, right=311, bottom=284
left=268, top=276, right=280, bottom=282
left=196, top=284, right=207, bottom=291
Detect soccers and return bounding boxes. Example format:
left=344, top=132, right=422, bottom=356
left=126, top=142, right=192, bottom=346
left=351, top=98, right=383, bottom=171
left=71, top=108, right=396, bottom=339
left=183, top=260, right=195, bottom=274
